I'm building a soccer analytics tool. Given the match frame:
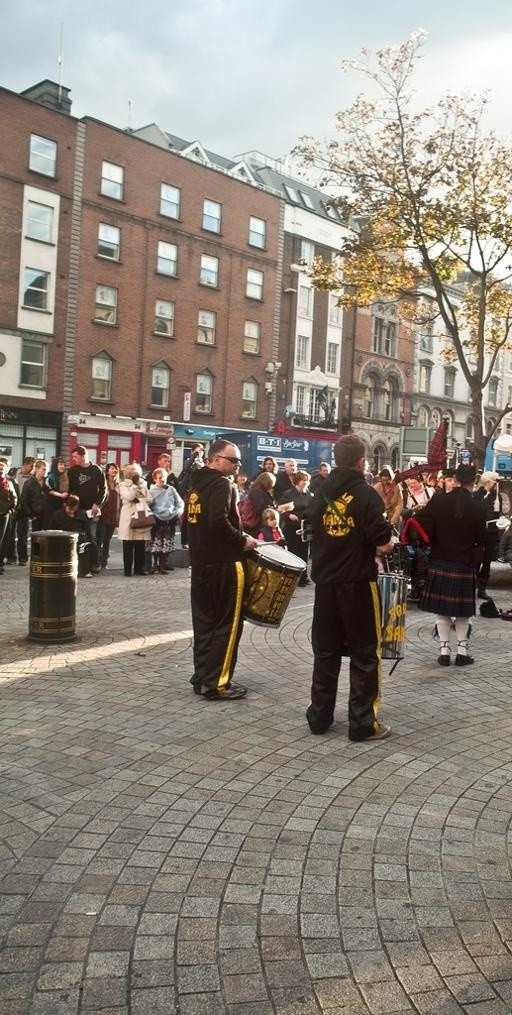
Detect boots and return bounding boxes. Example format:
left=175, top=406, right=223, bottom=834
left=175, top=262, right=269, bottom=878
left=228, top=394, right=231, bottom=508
left=478, top=573, right=492, bottom=600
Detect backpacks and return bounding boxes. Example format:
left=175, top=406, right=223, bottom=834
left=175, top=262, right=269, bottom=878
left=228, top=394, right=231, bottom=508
left=235, top=487, right=261, bottom=528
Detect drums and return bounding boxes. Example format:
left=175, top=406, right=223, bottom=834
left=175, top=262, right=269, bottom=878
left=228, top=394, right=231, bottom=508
left=242, top=541, right=307, bottom=628
left=376, top=572, right=409, bottom=676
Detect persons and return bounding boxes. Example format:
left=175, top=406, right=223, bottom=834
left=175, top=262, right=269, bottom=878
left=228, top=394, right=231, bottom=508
left=418, top=463, right=487, bottom=666
left=302, top=434, right=395, bottom=742
left=177, top=444, right=503, bottom=600
left=0, top=443, right=185, bottom=578
left=181, top=438, right=260, bottom=700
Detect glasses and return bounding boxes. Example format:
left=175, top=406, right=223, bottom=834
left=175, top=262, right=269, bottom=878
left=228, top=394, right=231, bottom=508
left=217, top=455, right=242, bottom=464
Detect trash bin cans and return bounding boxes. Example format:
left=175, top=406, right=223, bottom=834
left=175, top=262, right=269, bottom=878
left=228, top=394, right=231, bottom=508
left=26, top=529, right=80, bottom=644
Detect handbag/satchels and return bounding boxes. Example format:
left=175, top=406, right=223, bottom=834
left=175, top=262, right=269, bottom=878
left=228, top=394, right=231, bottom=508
left=130, top=508, right=157, bottom=529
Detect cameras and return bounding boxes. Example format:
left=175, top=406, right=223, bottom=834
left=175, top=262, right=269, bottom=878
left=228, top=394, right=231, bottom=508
left=201, top=448, right=206, bottom=452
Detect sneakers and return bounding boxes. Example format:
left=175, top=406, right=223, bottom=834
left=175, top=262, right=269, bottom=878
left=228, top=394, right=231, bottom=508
left=124, top=565, right=173, bottom=576
left=454, top=654, right=475, bottom=665
left=6, top=560, right=26, bottom=565
left=438, top=655, right=450, bottom=665
left=207, top=685, right=248, bottom=702
left=81, top=573, right=93, bottom=577
left=363, top=725, right=392, bottom=740
